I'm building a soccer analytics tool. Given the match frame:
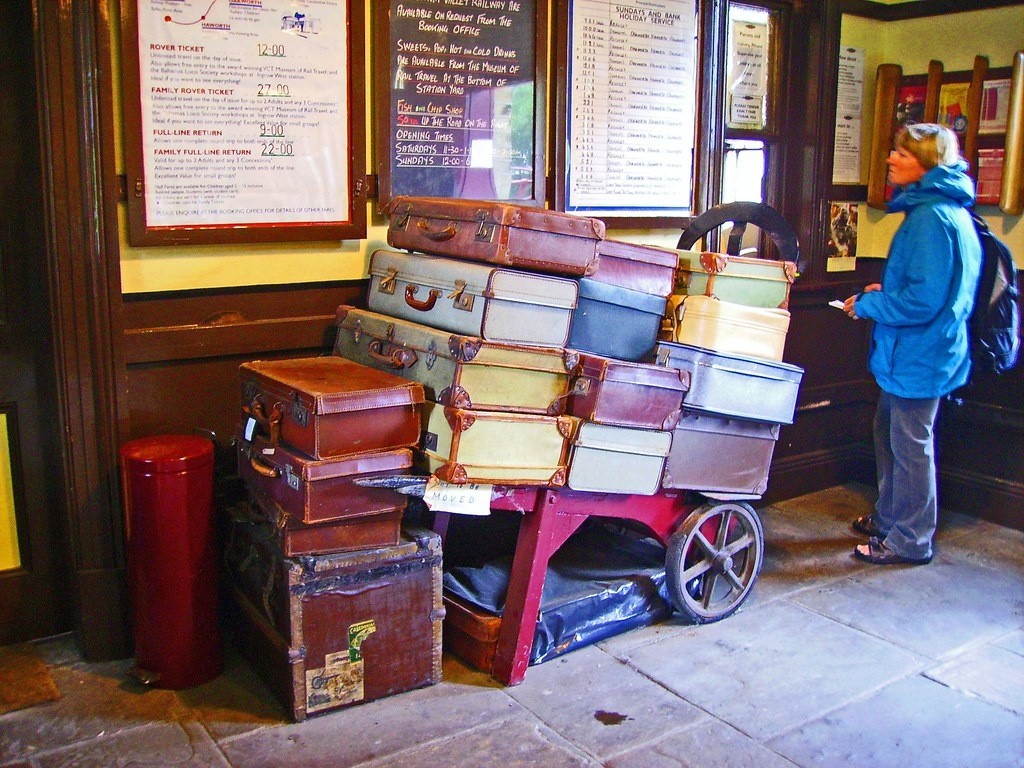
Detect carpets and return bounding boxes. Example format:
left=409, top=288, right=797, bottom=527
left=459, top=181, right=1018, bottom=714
left=1, top=643, right=62, bottom=715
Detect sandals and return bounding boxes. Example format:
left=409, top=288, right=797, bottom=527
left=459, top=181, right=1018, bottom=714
left=853, top=516, right=881, bottom=535
left=855, top=536, right=933, bottom=565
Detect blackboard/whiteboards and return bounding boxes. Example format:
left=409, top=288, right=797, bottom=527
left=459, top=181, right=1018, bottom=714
left=370, top=0, right=549, bottom=214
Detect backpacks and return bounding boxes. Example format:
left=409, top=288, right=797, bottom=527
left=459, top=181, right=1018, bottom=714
left=971, top=212, right=1021, bottom=374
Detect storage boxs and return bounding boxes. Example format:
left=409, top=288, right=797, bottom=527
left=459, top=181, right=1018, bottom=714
left=219, top=504, right=447, bottom=722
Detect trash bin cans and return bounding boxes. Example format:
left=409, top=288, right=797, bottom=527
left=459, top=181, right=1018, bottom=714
left=118, top=432, right=215, bottom=689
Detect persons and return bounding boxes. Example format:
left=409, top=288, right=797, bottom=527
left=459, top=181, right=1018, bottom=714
left=843, top=123, right=982, bottom=566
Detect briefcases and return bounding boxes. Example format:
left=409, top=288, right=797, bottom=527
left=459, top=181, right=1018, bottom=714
left=218, top=196, right=808, bottom=561
